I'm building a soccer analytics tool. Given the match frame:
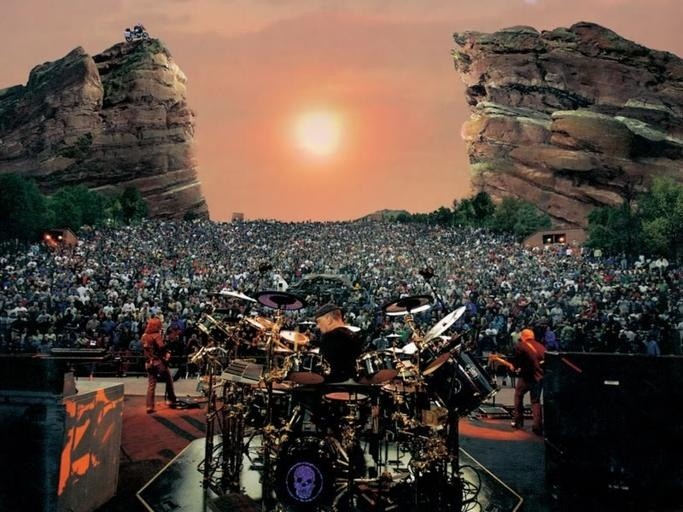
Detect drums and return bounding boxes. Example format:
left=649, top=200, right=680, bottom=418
left=234, top=317, right=274, bottom=349
left=270, top=330, right=309, bottom=373
left=359, top=351, right=397, bottom=381
left=423, top=342, right=496, bottom=417
left=321, top=392, right=371, bottom=438
left=196, top=312, right=240, bottom=354
left=378, top=383, right=427, bottom=442
left=276, top=437, right=340, bottom=509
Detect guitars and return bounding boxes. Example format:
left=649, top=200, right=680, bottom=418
left=487, top=354, right=521, bottom=376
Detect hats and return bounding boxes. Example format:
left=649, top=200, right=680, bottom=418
left=315, top=304, right=343, bottom=322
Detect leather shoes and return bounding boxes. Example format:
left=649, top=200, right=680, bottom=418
left=512, top=421, right=523, bottom=429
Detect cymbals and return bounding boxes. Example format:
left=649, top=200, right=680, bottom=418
left=286, top=372, right=325, bottom=385
left=423, top=306, right=466, bottom=340
left=384, top=295, right=433, bottom=315
left=324, top=378, right=371, bottom=388
left=207, top=290, right=258, bottom=305
left=252, top=291, right=306, bottom=309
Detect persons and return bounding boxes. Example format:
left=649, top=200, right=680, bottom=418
left=488, top=328, right=547, bottom=435
left=291, top=302, right=364, bottom=433
left=141, top=318, right=177, bottom=414
left=0, top=214, right=682, bottom=389
